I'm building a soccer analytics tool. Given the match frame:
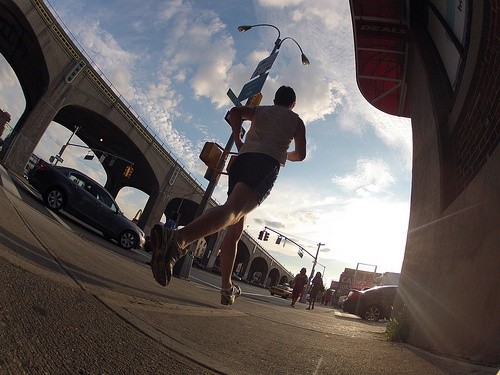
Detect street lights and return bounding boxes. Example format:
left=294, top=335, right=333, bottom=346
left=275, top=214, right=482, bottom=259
left=301, top=243, right=325, bottom=304
left=173, top=24, right=311, bottom=281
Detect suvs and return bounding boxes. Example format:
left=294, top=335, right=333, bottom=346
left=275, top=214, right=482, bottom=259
left=337, top=285, right=398, bottom=321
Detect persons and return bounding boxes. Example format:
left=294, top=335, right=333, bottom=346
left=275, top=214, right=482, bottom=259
left=291, top=268, right=307, bottom=306
left=153, top=87, right=306, bottom=304
left=306, top=271, right=323, bottom=311
left=322, top=294, right=332, bottom=305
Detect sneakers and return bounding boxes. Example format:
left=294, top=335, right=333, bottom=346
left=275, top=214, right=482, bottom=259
left=220, top=282, right=242, bottom=305
left=151, top=223, right=188, bottom=286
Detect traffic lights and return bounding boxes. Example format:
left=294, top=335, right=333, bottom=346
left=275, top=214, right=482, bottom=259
left=122, top=166, right=134, bottom=178
left=258, top=230, right=264, bottom=240
left=276, top=236, right=282, bottom=244
left=264, top=231, right=269, bottom=241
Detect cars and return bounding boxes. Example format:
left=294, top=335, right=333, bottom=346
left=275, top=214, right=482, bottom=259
left=191, top=256, right=266, bottom=289
left=269, top=283, right=293, bottom=299
left=26, top=159, right=146, bottom=250
